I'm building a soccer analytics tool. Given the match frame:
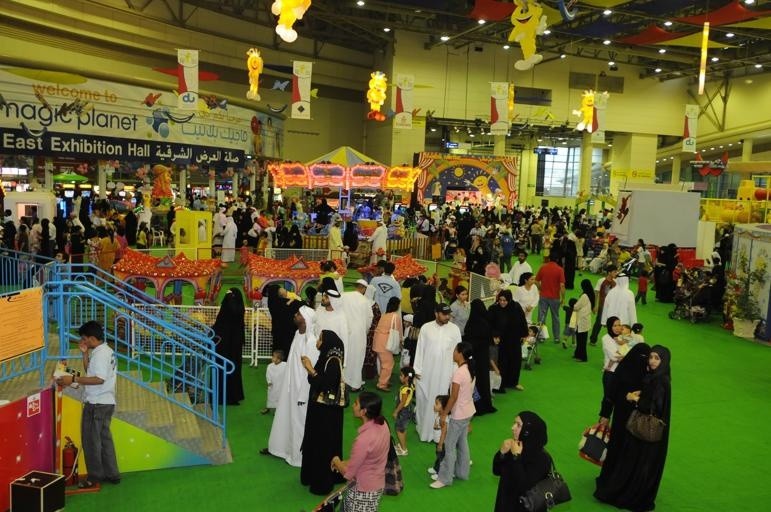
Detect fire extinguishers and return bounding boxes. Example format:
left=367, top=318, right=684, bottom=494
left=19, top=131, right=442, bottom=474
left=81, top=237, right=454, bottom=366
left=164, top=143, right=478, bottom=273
left=62, top=435, right=78, bottom=488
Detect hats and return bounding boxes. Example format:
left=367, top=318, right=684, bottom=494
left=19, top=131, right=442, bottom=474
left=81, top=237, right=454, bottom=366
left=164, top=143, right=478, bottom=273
left=374, top=259, right=386, bottom=266
left=631, top=323, right=643, bottom=332
left=352, top=278, right=368, bottom=287
left=434, top=303, right=453, bottom=314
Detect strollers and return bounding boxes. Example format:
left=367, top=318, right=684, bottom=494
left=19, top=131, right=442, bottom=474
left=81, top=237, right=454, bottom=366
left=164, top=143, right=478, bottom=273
left=167, top=348, right=204, bottom=405
left=668, top=290, right=698, bottom=324
left=311, top=480, right=356, bottom=512
left=521, top=311, right=546, bottom=370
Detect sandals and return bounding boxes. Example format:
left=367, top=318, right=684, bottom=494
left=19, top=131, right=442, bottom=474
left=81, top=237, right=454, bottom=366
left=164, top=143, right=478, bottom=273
left=260, top=447, right=270, bottom=455
left=78, top=480, right=94, bottom=489
left=103, top=478, right=121, bottom=484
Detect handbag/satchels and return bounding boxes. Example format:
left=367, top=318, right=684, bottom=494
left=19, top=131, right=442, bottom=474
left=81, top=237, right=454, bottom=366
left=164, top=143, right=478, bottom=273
left=625, top=403, right=666, bottom=453
left=315, top=355, right=350, bottom=408
left=518, top=452, right=572, bottom=512
left=580, top=420, right=607, bottom=461
left=385, top=314, right=400, bottom=354
left=383, top=417, right=404, bottom=496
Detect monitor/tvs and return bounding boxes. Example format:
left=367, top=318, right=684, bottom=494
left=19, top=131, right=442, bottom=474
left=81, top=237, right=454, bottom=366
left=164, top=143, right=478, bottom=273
left=131, top=198, right=137, bottom=204
left=60, top=188, right=77, bottom=201
left=79, top=188, right=93, bottom=199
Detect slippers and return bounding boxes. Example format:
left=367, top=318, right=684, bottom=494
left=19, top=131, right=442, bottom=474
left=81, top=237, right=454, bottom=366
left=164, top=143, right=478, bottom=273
left=351, top=387, right=364, bottom=392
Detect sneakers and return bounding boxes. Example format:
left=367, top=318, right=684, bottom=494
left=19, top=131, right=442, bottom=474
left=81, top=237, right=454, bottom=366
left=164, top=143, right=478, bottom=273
left=379, top=383, right=391, bottom=391
left=590, top=340, right=596, bottom=346
left=554, top=337, right=560, bottom=343
left=431, top=474, right=438, bottom=480
left=393, top=443, right=401, bottom=450
left=428, top=467, right=436, bottom=473
left=562, top=341, right=568, bottom=349
left=571, top=344, right=576, bottom=348
left=429, top=479, right=445, bottom=488
left=261, top=407, right=269, bottom=413
left=376, top=383, right=392, bottom=390
left=395, top=446, right=408, bottom=456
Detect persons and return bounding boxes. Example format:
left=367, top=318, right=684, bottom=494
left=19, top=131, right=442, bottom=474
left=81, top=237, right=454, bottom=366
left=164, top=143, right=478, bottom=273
left=487, top=289, right=530, bottom=392
left=18, top=216, right=31, bottom=234
left=2, top=208, right=14, bottom=224
left=632, top=239, right=649, bottom=276
left=278, top=288, right=303, bottom=305
left=603, top=325, right=633, bottom=371
left=16, top=225, right=31, bottom=257
left=511, top=272, right=540, bottom=324
left=343, top=221, right=359, bottom=253
left=573, top=279, right=596, bottom=363
left=521, top=326, right=538, bottom=359
left=300, top=331, right=346, bottom=491
left=145, top=228, right=154, bottom=249
left=431, top=342, right=477, bottom=488
left=210, top=287, right=245, bottom=406
left=113, top=227, right=128, bottom=263
left=654, top=246, right=667, bottom=269
left=373, top=258, right=386, bottom=275
left=255, top=209, right=270, bottom=228
left=45, top=219, right=56, bottom=250
left=628, top=344, right=671, bottom=512
left=354, top=201, right=372, bottom=218
left=54, top=253, right=68, bottom=265
left=221, top=216, right=239, bottom=263
left=317, top=261, right=341, bottom=282
left=99, top=210, right=107, bottom=227
left=635, top=271, right=649, bottom=304
left=561, top=298, right=578, bottom=349
left=365, top=217, right=386, bottom=265
left=260, top=351, right=287, bottom=414
left=493, top=411, right=553, bottom=512
left=589, top=265, right=618, bottom=346
left=92, top=208, right=103, bottom=225
left=601, top=273, right=637, bottom=325
left=315, top=289, right=346, bottom=339
left=166, top=205, right=177, bottom=228
left=331, top=392, right=390, bottom=512
left=630, top=323, right=646, bottom=341
left=225, top=202, right=235, bottom=215
left=95, top=228, right=120, bottom=285
left=392, top=367, right=415, bottom=456
left=593, top=341, right=649, bottom=508
left=342, top=245, right=351, bottom=268
left=109, top=213, right=120, bottom=226
left=619, top=247, right=630, bottom=266
left=694, top=272, right=719, bottom=308
left=498, top=251, right=532, bottom=285
left=285, top=226, right=303, bottom=249
left=533, top=251, right=565, bottom=344
left=191, top=196, right=207, bottom=211
left=246, top=217, right=262, bottom=250
left=303, top=285, right=317, bottom=307
left=395, top=278, right=419, bottom=314
left=413, top=302, right=462, bottom=445
left=259, top=306, right=319, bottom=466
left=362, top=285, right=378, bottom=379
left=340, top=279, right=374, bottom=392
left=608, top=238, right=620, bottom=266
left=372, top=296, right=403, bottom=391
left=262, top=285, right=297, bottom=360
left=325, top=213, right=344, bottom=261
left=463, top=300, right=497, bottom=416
left=599, top=318, right=625, bottom=385
left=269, top=191, right=335, bottom=223
left=401, top=314, right=418, bottom=367
left=56, top=320, right=122, bottom=489
left=328, top=258, right=344, bottom=290
left=415, top=201, right=613, bottom=252
left=314, top=277, right=339, bottom=301
left=32, top=218, right=42, bottom=249
left=367, top=261, right=401, bottom=311
left=712, top=258, right=725, bottom=284
left=136, top=221, right=147, bottom=251
left=68, top=225, right=86, bottom=246
left=124, top=210, right=138, bottom=243
left=446, top=285, right=471, bottom=329
left=68, top=210, right=85, bottom=233
left=666, top=243, right=679, bottom=268
left=428, top=397, right=450, bottom=480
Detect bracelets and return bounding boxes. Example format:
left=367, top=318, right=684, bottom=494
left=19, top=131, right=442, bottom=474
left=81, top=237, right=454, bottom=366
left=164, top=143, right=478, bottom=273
left=72, top=375, right=76, bottom=382
left=312, top=371, right=318, bottom=376
